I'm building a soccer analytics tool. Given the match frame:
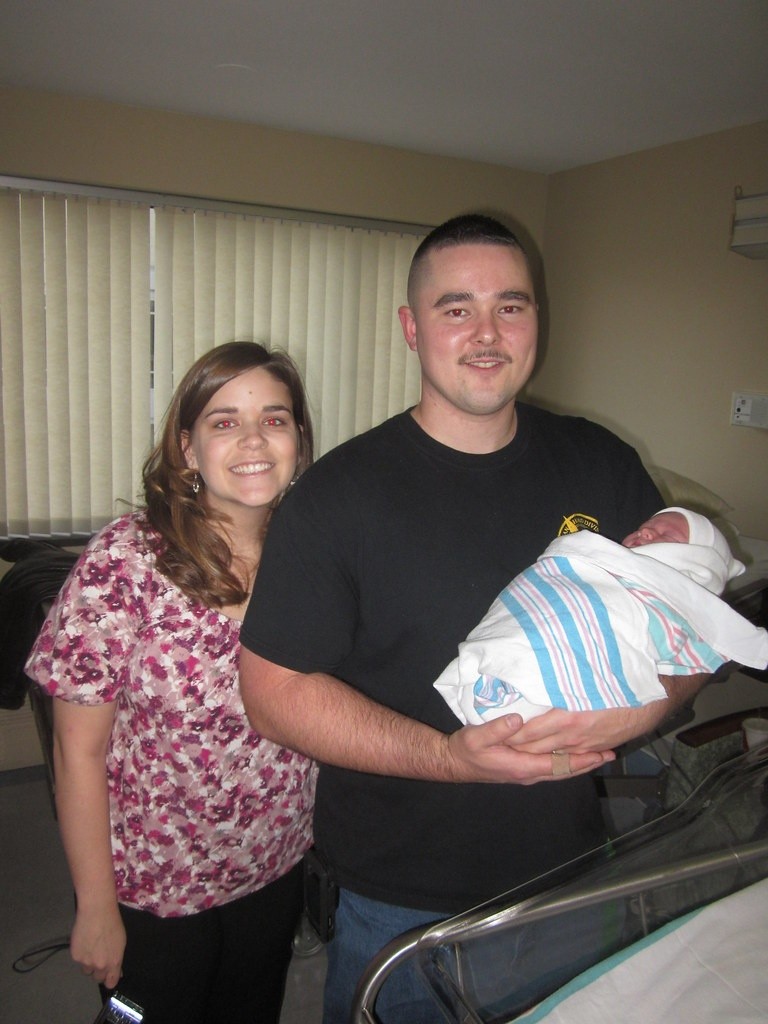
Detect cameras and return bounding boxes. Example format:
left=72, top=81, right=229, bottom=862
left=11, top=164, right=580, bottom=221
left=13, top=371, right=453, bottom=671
left=93, top=991, right=144, bottom=1024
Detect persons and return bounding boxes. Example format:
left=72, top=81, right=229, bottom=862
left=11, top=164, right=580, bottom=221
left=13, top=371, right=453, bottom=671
left=235, top=214, right=711, bottom=1024
left=24, top=341, right=321, bottom=1024
left=434, top=506, right=768, bottom=727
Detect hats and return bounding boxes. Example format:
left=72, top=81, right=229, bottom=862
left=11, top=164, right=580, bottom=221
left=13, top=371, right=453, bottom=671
left=649, top=506, right=746, bottom=582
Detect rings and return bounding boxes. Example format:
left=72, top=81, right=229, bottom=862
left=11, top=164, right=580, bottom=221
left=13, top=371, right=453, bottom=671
left=552, top=751, right=571, bottom=778
